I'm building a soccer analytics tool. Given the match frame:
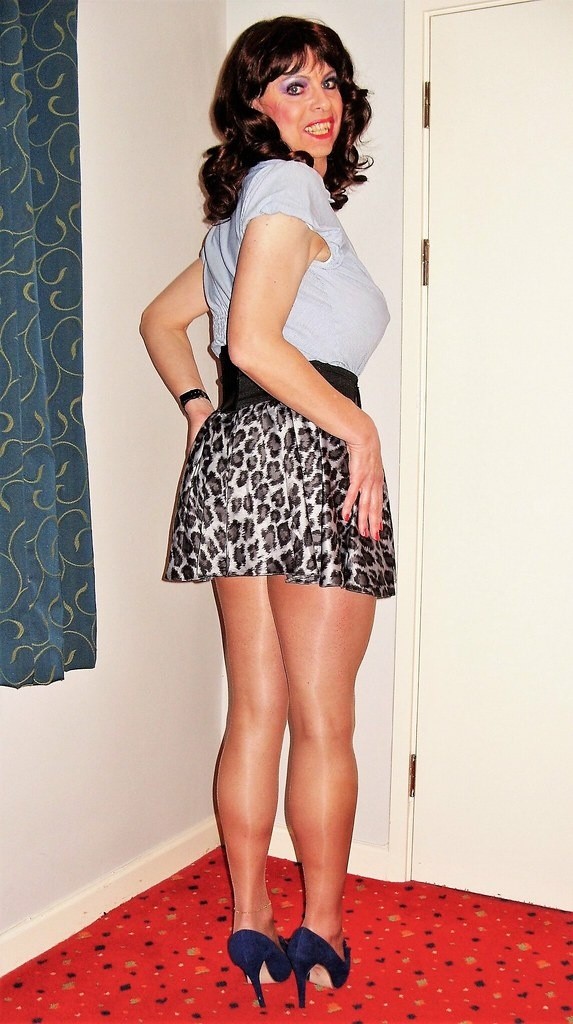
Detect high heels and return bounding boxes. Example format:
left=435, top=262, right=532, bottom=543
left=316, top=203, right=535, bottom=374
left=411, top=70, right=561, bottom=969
left=227, top=929, right=292, bottom=1008
left=287, top=927, right=351, bottom=1008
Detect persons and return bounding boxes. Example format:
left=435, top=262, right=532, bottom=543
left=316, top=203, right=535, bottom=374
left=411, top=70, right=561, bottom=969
left=138, top=16, right=397, bottom=1007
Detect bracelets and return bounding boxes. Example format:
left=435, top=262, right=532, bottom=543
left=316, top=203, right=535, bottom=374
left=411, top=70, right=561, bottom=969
left=179, top=389, right=211, bottom=415
